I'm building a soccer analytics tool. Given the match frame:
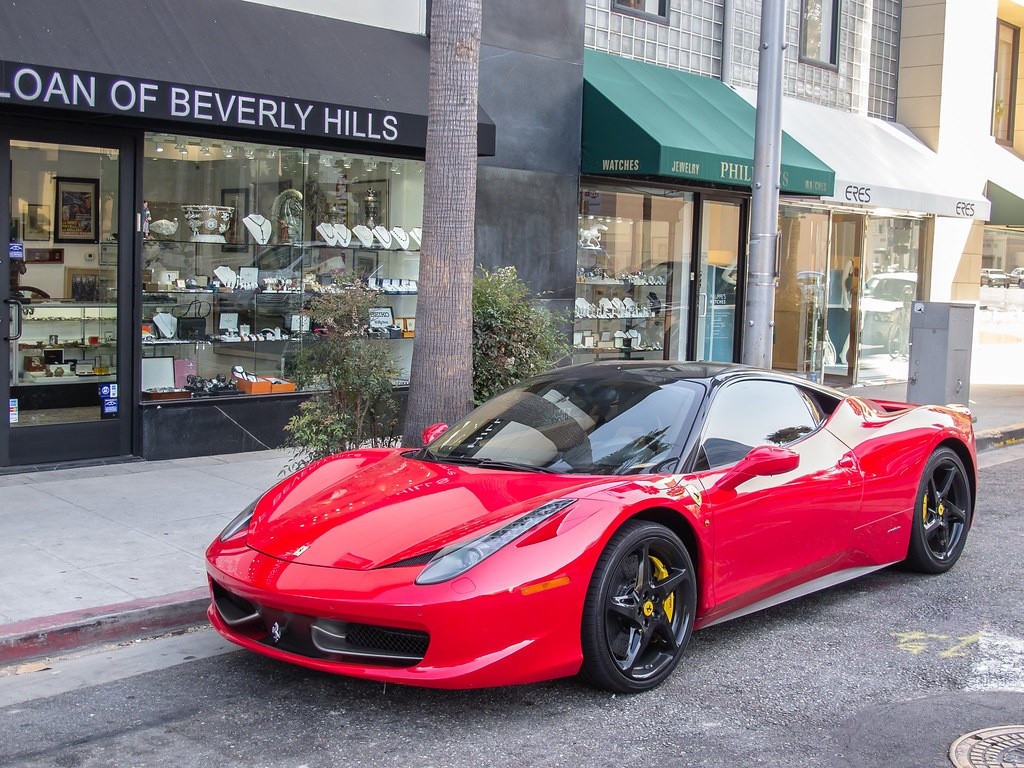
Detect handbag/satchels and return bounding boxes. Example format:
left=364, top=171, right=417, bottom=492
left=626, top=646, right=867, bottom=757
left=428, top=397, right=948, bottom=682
left=177, top=317, right=206, bottom=341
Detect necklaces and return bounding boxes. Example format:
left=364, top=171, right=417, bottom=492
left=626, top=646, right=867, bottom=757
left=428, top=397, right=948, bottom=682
left=628, top=331, right=638, bottom=338
left=159, top=314, right=172, bottom=334
left=372, top=284, right=405, bottom=291
left=247, top=215, right=422, bottom=244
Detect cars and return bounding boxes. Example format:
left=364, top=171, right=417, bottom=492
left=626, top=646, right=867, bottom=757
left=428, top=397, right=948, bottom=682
left=796, top=271, right=827, bottom=294
left=862, top=272, right=918, bottom=344
left=980, top=269, right=1011, bottom=289
left=1009, top=267, right=1024, bottom=287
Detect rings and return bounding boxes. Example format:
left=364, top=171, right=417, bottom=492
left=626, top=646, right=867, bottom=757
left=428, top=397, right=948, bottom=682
left=229, top=333, right=262, bottom=338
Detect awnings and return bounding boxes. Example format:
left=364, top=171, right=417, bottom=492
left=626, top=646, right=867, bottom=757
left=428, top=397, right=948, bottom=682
left=987, top=144, right=1024, bottom=226
left=581, top=48, right=834, bottom=201
left=732, top=82, right=995, bottom=220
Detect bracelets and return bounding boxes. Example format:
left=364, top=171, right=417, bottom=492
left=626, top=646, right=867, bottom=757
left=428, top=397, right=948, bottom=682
left=647, top=276, right=654, bottom=283
left=656, top=275, right=663, bottom=284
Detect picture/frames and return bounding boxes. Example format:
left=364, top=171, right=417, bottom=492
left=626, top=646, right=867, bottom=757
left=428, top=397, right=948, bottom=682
left=221, top=187, right=249, bottom=253
left=345, top=178, right=390, bottom=242
left=53, top=176, right=99, bottom=244
left=23, top=203, right=51, bottom=241
left=353, top=249, right=378, bottom=286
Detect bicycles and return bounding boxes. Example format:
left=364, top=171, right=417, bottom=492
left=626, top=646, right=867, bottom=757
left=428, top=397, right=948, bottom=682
left=884, top=307, right=907, bottom=359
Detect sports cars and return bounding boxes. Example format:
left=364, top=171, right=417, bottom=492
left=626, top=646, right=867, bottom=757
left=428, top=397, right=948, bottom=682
left=208, top=356, right=979, bottom=693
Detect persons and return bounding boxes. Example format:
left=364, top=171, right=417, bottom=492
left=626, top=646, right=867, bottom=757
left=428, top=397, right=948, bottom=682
left=142, top=200, right=151, bottom=237
left=840, top=257, right=872, bottom=364
left=63, top=193, right=91, bottom=231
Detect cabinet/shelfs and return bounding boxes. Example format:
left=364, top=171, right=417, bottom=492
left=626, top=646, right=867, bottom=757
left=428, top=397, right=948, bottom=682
left=573, top=270, right=666, bottom=355
left=16, top=230, right=420, bottom=388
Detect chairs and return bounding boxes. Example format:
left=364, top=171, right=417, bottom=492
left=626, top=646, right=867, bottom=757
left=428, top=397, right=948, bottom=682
left=617, top=386, right=661, bottom=426
